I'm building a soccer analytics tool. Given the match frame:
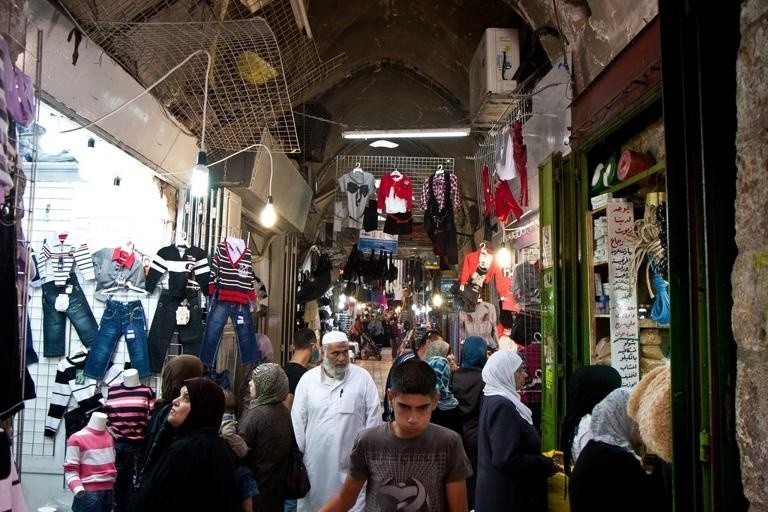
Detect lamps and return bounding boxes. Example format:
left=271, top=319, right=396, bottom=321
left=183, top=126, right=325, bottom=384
left=256, top=195, right=277, bottom=229
left=188, top=150, right=213, bottom=195
left=340, top=127, right=476, bottom=139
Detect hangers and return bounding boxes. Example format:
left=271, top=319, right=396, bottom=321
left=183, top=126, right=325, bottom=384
left=436, top=164, right=444, bottom=176
left=70, top=337, right=90, bottom=359
left=390, top=167, right=402, bottom=180
left=120, top=242, right=136, bottom=265
left=532, top=331, right=543, bottom=348
left=353, top=161, right=364, bottom=174
left=100, top=281, right=150, bottom=297
left=519, top=368, right=542, bottom=393
left=159, top=277, right=203, bottom=291
left=175, top=231, right=190, bottom=248
left=477, top=242, right=489, bottom=255
left=50, top=230, right=75, bottom=249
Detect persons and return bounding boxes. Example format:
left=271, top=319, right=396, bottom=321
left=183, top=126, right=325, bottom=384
left=316, top=360, right=474, bottom=512
left=424, top=356, right=463, bottom=437
left=105, top=368, right=155, bottom=510
left=213, top=388, right=258, bottom=512
left=239, top=361, right=297, bottom=512
left=276, top=327, right=314, bottom=511
left=66, top=410, right=116, bottom=509
left=422, top=339, right=456, bottom=373
left=382, top=332, right=445, bottom=426
left=473, top=350, right=565, bottom=511
left=452, top=335, right=490, bottom=512
left=138, top=376, right=240, bottom=512
left=558, top=361, right=623, bottom=499
left=290, top=331, right=382, bottom=511
left=565, top=386, right=667, bottom=511
left=350, top=309, right=400, bottom=359
left=486, top=345, right=493, bottom=362
left=151, top=352, right=203, bottom=425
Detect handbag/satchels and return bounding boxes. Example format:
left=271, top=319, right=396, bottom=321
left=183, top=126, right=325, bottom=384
left=284, top=448, right=311, bottom=500
left=389, top=264, right=397, bottom=280
left=369, top=259, right=379, bottom=279
left=377, top=260, right=388, bottom=277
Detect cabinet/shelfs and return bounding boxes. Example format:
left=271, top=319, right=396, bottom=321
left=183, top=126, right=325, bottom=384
left=585, top=103, right=669, bottom=389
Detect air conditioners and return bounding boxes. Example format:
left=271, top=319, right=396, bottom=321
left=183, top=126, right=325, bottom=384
left=466, top=27, right=519, bottom=126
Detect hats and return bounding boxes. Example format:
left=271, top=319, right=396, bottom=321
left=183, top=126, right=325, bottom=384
left=322, top=331, right=348, bottom=345
